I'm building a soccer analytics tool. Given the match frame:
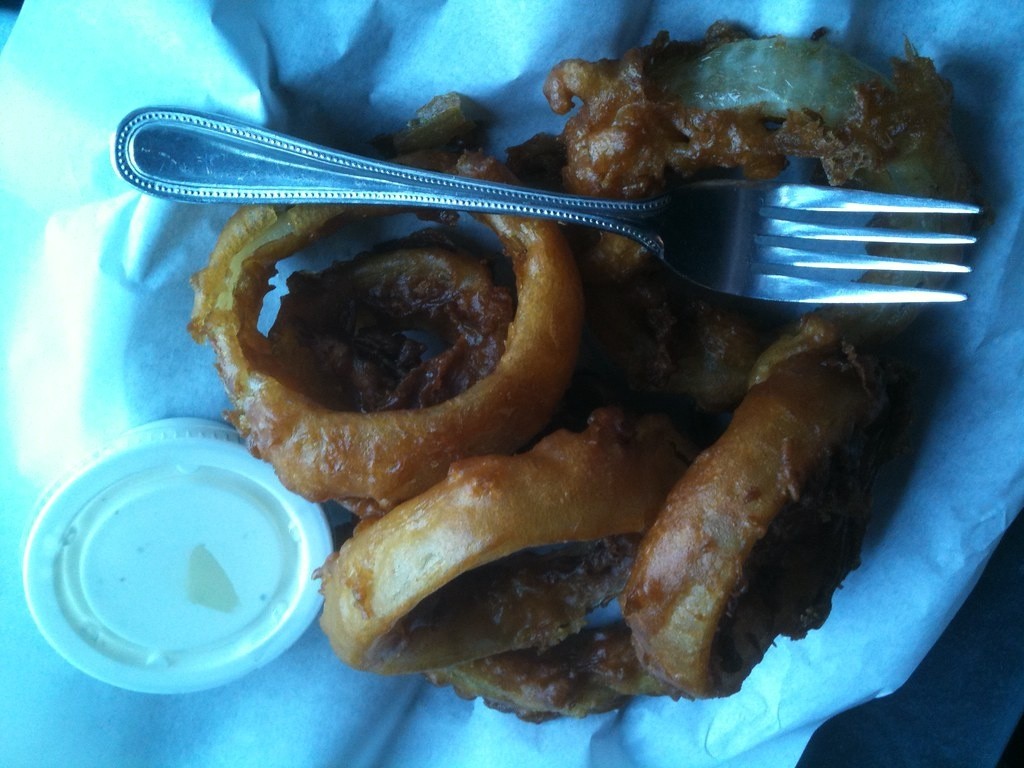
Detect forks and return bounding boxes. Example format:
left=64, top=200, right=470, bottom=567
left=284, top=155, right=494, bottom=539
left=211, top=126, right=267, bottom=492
left=111, top=105, right=981, bottom=305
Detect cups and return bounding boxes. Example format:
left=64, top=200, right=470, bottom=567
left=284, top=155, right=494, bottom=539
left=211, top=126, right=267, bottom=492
left=20, top=417, right=335, bottom=695
